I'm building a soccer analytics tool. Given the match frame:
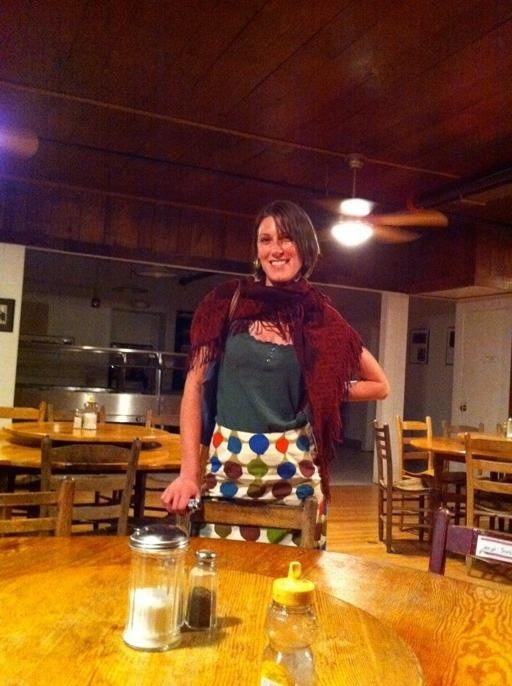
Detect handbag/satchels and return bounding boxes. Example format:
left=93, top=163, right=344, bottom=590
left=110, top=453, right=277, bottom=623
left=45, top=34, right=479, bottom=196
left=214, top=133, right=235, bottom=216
left=199, top=278, right=242, bottom=447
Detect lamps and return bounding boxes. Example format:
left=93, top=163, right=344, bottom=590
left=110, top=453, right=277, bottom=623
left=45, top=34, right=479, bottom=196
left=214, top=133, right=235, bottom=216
left=328, top=197, right=376, bottom=246
left=138, top=266, right=177, bottom=278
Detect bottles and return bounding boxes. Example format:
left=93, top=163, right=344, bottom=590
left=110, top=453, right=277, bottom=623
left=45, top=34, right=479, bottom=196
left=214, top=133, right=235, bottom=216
left=264, top=561, right=318, bottom=655
left=72, top=430, right=82, bottom=438
left=257, top=641, right=317, bottom=686
left=72, top=408, right=83, bottom=429
left=81, top=402, right=100, bottom=430
left=502, top=417, right=512, bottom=439
left=185, top=546, right=219, bottom=632
left=121, top=524, right=193, bottom=653
left=82, top=428, right=97, bottom=439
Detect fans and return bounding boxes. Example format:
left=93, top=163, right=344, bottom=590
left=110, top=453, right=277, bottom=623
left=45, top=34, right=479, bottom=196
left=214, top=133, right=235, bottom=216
left=314, top=151, right=446, bottom=242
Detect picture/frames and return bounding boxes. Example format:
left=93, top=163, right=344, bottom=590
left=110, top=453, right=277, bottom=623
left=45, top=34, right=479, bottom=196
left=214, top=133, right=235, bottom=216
left=446, top=327, right=455, bottom=365
left=0, top=298, right=15, bottom=332
left=408, top=329, right=429, bottom=364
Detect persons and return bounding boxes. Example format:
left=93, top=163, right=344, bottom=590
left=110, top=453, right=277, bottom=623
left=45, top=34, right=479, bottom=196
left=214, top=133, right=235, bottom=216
left=160, top=198, right=391, bottom=551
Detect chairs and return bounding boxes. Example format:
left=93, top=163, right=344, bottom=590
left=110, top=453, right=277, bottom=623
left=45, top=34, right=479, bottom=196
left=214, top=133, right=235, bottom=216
left=373, top=415, right=512, bottom=575
